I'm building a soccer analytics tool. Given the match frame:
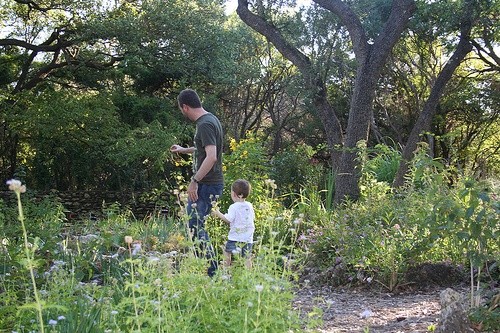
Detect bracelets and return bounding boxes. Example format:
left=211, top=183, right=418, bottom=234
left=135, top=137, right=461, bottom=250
left=190, top=176, right=199, bottom=184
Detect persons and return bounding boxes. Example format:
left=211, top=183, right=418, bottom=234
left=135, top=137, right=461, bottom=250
left=211, top=179, right=255, bottom=268
left=169, top=90, right=224, bottom=278
